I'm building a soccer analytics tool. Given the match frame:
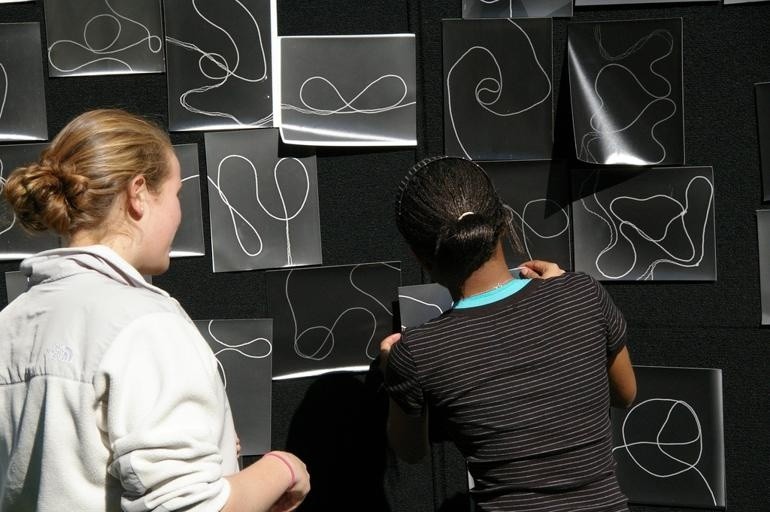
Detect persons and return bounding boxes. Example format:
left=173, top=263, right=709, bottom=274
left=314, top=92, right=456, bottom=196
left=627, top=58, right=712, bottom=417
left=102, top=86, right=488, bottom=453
left=1, top=105, right=313, bottom=511
left=362, top=153, right=639, bottom=510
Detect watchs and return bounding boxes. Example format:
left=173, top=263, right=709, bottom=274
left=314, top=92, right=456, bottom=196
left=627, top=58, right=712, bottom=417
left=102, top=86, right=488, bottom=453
left=262, top=450, right=296, bottom=487
